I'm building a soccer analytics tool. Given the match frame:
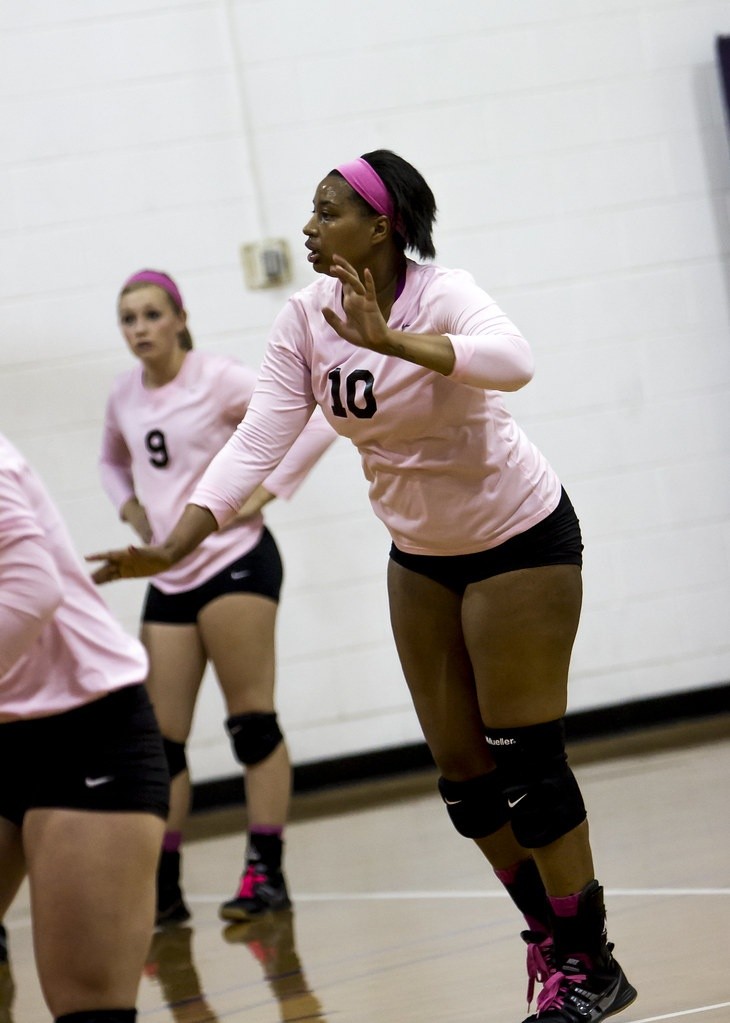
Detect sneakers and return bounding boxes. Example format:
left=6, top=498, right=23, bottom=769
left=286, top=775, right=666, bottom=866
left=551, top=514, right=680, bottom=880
left=518, top=916, right=637, bottom=1023
left=152, top=881, right=191, bottom=923
left=143, top=927, right=195, bottom=974
left=247, top=935, right=292, bottom=970
left=221, top=861, right=291, bottom=921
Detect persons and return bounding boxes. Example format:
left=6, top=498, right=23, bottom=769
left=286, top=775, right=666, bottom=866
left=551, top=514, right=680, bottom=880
left=83, top=150, right=638, bottom=1023
left=0, top=427, right=173, bottom=1022
left=96, top=268, right=293, bottom=927
